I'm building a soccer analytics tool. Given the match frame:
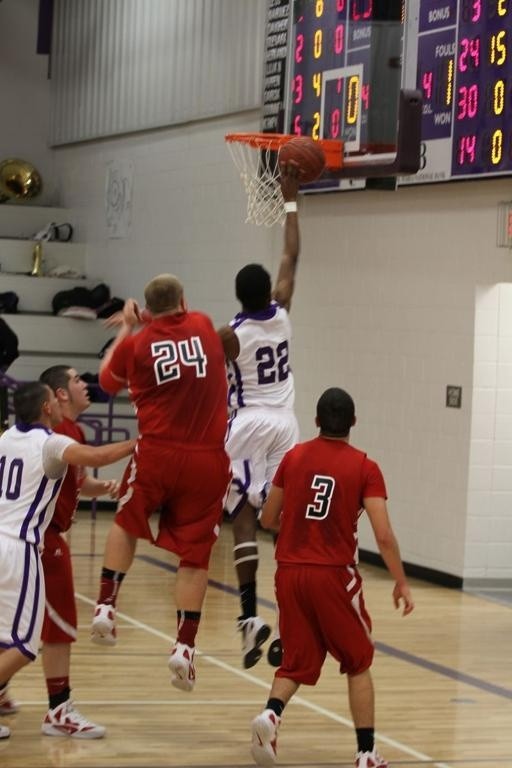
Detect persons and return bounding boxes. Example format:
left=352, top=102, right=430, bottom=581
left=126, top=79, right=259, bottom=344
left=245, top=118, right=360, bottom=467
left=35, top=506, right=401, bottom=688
left=252, top=387, right=413, bottom=767
left=218, top=159, right=306, bottom=667
left=88, top=275, right=230, bottom=693
left=1, top=316, right=19, bottom=432
left=1, top=383, right=137, bottom=739
left=38, top=368, right=119, bottom=741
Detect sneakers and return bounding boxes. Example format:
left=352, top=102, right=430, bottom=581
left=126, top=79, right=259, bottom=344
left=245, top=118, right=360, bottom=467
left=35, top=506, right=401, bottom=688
left=168, top=641, right=197, bottom=692
left=353, top=744, right=389, bottom=768
left=41, top=699, right=107, bottom=740
left=89, top=603, right=119, bottom=647
left=236, top=615, right=272, bottom=670
left=0, top=683, right=21, bottom=739
left=249, top=708, right=283, bottom=768
left=266, top=635, right=282, bottom=667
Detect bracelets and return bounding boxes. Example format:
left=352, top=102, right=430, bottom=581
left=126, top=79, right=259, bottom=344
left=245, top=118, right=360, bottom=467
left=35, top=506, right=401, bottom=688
left=283, top=201, right=298, bottom=214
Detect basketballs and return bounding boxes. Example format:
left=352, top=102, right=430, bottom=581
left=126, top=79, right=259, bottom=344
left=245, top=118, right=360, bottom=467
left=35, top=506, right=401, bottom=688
left=277, top=136, right=325, bottom=184
